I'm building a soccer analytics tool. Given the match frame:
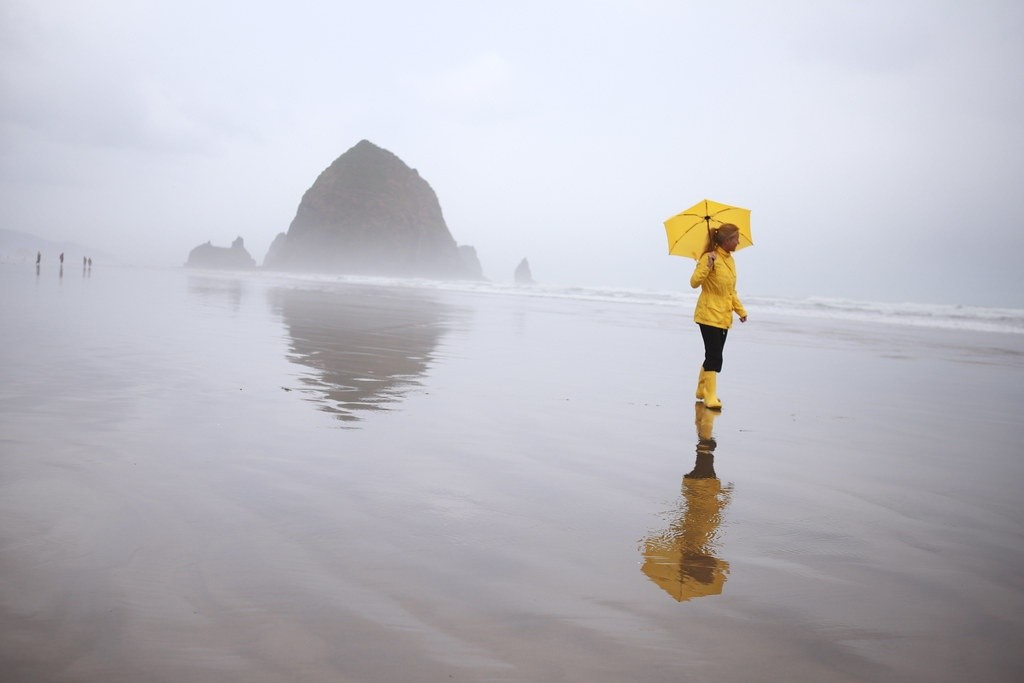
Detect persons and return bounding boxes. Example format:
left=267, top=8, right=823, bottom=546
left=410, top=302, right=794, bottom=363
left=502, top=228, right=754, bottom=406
left=667, top=401, right=734, bottom=585
left=34, top=248, right=93, bottom=280
left=690, top=223, right=747, bottom=409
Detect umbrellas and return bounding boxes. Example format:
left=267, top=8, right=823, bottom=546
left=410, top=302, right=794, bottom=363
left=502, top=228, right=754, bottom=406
left=663, top=197, right=755, bottom=271
left=638, top=534, right=731, bottom=603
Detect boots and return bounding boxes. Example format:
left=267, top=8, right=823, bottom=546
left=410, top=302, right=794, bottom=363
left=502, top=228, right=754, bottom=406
left=703, top=371, right=722, bottom=409
left=696, top=365, right=721, bottom=402
